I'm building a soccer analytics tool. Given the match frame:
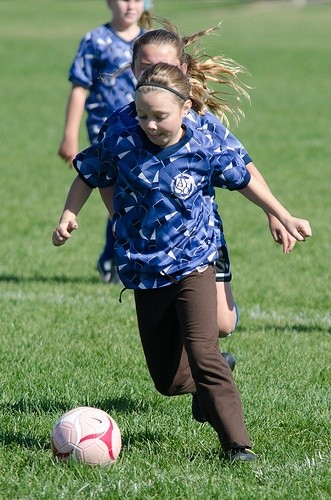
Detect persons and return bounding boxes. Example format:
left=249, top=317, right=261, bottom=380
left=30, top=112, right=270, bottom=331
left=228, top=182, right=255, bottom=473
left=57, top=0, right=155, bottom=288
left=91, top=29, right=297, bottom=338
left=52, top=62, right=314, bottom=464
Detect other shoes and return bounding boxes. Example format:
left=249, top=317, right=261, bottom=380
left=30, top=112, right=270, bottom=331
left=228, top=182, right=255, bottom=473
left=227, top=448, right=258, bottom=461
left=191, top=353, right=236, bottom=423
left=98, top=255, right=118, bottom=284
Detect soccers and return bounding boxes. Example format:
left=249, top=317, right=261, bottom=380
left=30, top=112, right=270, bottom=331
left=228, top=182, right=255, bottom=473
left=51, top=406, right=121, bottom=468
